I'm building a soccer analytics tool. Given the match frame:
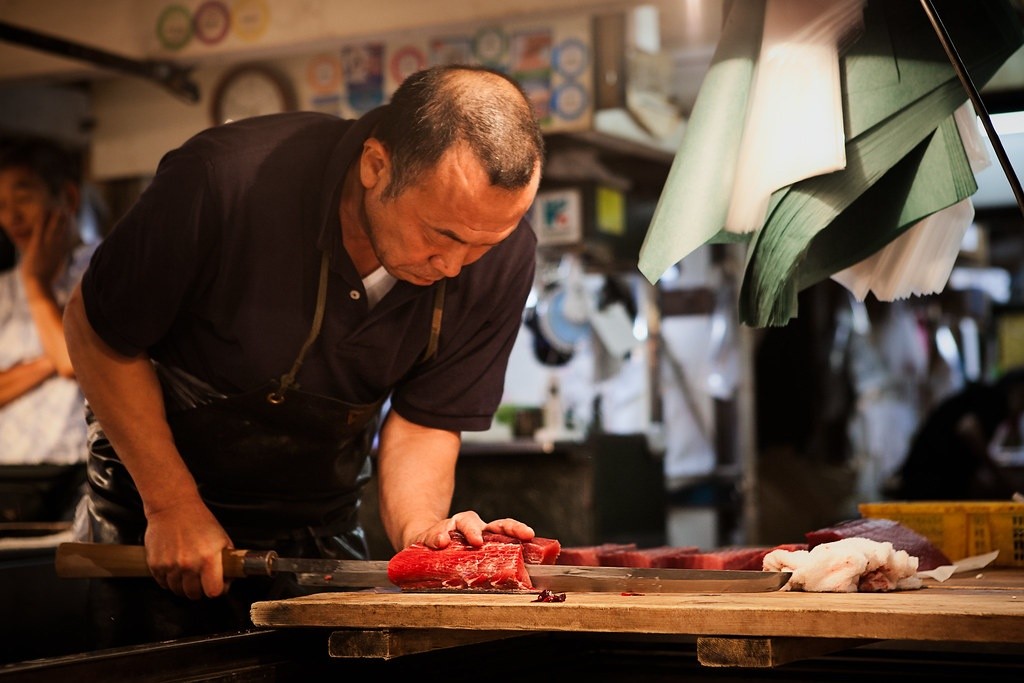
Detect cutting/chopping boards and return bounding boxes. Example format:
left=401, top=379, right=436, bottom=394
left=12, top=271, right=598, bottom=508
left=249, top=571, right=1024, bottom=642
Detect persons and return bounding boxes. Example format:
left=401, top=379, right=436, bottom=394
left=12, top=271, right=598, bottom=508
left=51, top=63, right=548, bottom=650
left=0, top=133, right=106, bottom=542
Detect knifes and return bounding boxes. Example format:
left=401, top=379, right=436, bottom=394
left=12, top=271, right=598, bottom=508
left=57, top=542, right=793, bottom=593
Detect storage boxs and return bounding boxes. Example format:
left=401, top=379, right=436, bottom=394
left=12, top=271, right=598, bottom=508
left=858, top=501, right=1024, bottom=568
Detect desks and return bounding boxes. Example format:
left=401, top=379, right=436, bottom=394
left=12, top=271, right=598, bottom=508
left=249, top=570, right=1024, bottom=674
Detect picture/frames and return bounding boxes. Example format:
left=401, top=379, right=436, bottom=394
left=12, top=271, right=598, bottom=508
left=535, top=189, right=582, bottom=244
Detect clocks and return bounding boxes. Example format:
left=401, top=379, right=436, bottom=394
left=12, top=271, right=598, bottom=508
left=207, top=59, right=299, bottom=126
